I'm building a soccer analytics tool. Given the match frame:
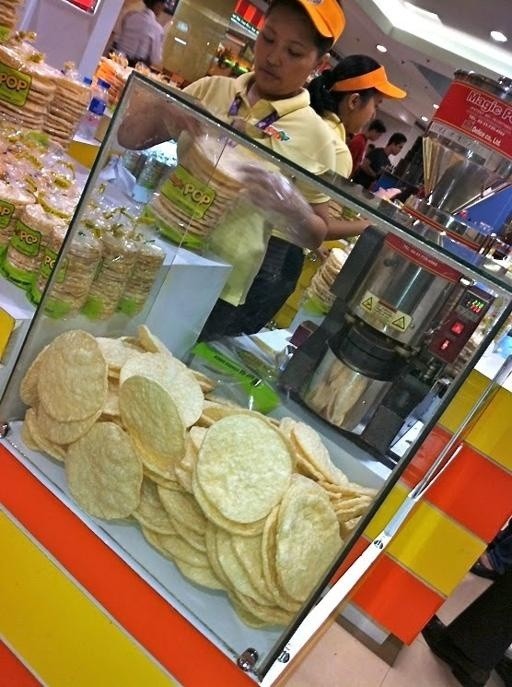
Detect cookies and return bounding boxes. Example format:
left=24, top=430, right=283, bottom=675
left=0, top=0, right=252, bottom=319
left=309, top=247, right=352, bottom=302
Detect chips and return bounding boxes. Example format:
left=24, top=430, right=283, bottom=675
left=19, top=325, right=377, bottom=629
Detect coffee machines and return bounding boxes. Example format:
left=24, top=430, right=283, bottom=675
left=283, top=63, right=511, bottom=455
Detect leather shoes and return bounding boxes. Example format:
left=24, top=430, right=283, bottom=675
left=495, top=655, right=512, bottom=687
left=469, top=555, right=502, bottom=581
left=421, top=613, right=490, bottom=687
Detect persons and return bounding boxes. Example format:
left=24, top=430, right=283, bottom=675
left=117, top=1, right=346, bottom=345
left=108, top=0, right=165, bottom=70
left=343, top=119, right=407, bottom=188
left=227, top=53, right=408, bottom=337
left=421, top=516, right=512, bottom=687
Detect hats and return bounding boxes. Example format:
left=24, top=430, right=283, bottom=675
left=295, top=0, right=346, bottom=49
left=326, top=62, right=407, bottom=102
